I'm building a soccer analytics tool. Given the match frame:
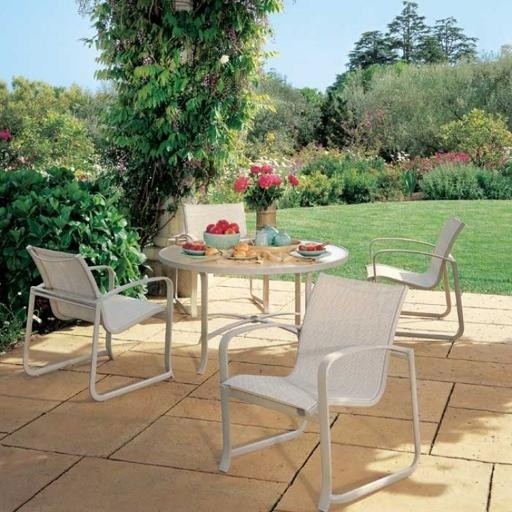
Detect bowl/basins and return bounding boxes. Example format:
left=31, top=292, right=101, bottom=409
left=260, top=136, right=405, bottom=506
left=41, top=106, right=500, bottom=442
left=203, top=231, right=240, bottom=249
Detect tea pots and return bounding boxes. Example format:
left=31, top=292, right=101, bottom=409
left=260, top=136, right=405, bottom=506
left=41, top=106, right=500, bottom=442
left=255, top=224, right=279, bottom=244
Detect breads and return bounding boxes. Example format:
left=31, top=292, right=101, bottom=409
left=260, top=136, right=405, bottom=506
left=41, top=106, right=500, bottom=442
left=205, top=243, right=256, bottom=257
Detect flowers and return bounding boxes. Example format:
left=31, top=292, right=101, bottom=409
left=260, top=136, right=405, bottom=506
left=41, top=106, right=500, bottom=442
left=235, top=163, right=299, bottom=212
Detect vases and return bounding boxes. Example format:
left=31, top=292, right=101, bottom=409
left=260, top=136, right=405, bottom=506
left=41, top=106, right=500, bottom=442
left=255, top=200, right=277, bottom=237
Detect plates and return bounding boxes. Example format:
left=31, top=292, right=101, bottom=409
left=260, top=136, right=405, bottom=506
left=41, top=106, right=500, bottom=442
left=226, top=250, right=259, bottom=260
left=180, top=248, right=222, bottom=261
left=296, top=246, right=327, bottom=257
left=182, top=246, right=206, bottom=256
left=240, top=237, right=301, bottom=254
left=290, top=250, right=332, bottom=261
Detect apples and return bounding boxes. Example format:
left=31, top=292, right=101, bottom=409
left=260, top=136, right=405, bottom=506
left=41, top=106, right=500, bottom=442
left=183, top=240, right=205, bottom=251
left=207, top=220, right=239, bottom=235
left=299, top=244, right=324, bottom=251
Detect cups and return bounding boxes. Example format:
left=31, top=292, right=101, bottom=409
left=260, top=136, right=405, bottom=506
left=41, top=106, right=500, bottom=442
left=274, top=233, right=291, bottom=246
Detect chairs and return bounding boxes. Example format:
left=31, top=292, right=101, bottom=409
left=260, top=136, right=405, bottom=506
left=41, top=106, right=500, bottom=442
left=217, top=271, right=421, bottom=512
left=22, top=245, right=175, bottom=402
left=365, top=216, right=465, bottom=340
left=174, top=201, right=270, bottom=320
